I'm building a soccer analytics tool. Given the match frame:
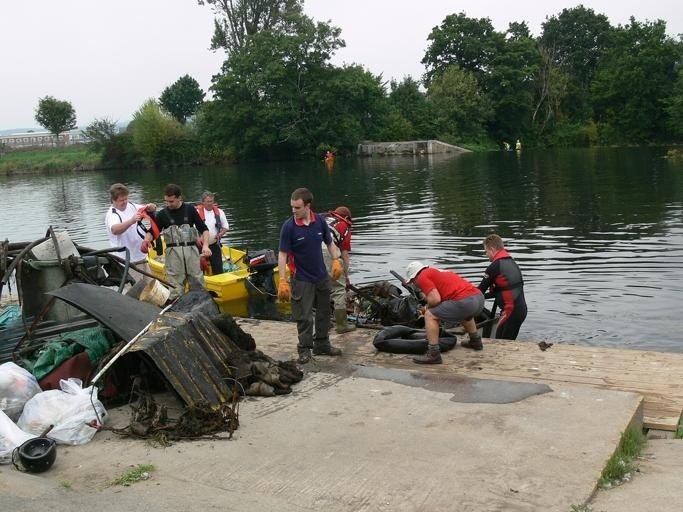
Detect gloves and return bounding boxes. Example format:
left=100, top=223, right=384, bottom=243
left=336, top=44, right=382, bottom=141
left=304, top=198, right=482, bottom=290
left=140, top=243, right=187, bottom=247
left=331, top=258, right=343, bottom=281
left=140, top=240, right=151, bottom=253
left=277, top=278, right=290, bottom=303
left=199, top=255, right=209, bottom=273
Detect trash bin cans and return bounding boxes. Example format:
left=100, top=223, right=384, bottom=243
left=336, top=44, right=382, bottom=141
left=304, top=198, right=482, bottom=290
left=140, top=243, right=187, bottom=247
left=15, top=256, right=109, bottom=321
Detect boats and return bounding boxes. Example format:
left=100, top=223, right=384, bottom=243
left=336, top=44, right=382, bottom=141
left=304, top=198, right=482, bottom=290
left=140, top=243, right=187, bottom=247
left=324, top=156, right=335, bottom=161
left=329, top=284, right=504, bottom=340
left=326, top=160, right=333, bottom=177
left=142, top=231, right=293, bottom=314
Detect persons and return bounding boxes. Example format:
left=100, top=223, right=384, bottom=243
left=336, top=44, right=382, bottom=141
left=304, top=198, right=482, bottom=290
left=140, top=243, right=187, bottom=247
left=273, top=187, right=344, bottom=364
left=140, top=183, right=213, bottom=300
left=501, top=140, right=511, bottom=152
left=194, top=189, right=229, bottom=275
left=324, top=151, right=333, bottom=161
left=404, top=260, right=486, bottom=364
left=104, top=182, right=157, bottom=283
left=286, top=205, right=357, bottom=334
left=514, top=139, right=521, bottom=150
left=475, top=233, right=528, bottom=340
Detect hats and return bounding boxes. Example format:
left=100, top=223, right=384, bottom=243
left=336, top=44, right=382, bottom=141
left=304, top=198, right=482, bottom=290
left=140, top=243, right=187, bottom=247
left=404, top=261, right=429, bottom=284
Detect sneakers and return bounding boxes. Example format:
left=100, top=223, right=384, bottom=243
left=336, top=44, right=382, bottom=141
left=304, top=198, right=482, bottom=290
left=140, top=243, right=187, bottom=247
left=299, top=356, right=310, bottom=363
left=321, top=346, right=342, bottom=356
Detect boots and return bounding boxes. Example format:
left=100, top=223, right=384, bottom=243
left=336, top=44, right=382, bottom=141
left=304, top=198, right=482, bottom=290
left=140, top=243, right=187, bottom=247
left=461, top=337, right=483, bottom=349
left=413, top=348, right=442, bottom=364
left=335, top=309, right=356, bottom=334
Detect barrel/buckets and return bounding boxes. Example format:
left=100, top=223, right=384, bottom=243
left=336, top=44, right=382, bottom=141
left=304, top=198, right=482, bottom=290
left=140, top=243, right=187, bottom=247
left=139, top=279, right=170, bottom=306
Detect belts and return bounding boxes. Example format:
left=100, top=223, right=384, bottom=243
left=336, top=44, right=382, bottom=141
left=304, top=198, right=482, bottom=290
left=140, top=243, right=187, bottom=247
left=167, top=242, right=195, bottom=247
